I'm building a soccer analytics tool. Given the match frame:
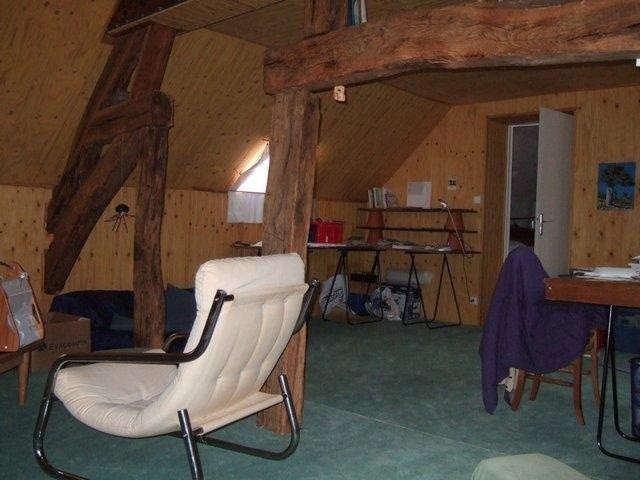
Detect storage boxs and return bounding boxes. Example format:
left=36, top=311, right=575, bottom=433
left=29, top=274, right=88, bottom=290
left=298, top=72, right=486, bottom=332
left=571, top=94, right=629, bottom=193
left=31, top=312, right=91, bottom=374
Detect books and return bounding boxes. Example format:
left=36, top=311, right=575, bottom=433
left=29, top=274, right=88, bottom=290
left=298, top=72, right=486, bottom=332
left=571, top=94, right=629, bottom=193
left=367, top=189, right=375, bottom=210
left=569, top=263, right=609, bottom=277
left=391, top=243, right=424, bottom=251
left=379, top=188, right=399, bottom=209
left=372, top=187, right=382, bottom=209
left=406, top=181, right=432, bottom=210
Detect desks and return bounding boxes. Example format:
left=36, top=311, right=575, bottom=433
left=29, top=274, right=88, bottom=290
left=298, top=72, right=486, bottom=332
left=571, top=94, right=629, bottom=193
left=544, top=274, right=640, bottom=465
left=309, top=243, right=481, bottom=330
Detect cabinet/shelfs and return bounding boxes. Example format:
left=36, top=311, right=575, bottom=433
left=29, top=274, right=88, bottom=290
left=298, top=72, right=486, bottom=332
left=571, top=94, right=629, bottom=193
left=355, top=206, right=478, bottom=251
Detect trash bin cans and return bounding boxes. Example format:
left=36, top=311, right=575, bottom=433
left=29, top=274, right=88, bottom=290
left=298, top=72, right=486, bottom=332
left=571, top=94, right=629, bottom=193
left=629, top=358, right=640, bottom=437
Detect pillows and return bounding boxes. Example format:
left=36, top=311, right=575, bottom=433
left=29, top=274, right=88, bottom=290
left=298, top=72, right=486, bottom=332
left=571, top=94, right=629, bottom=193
left=164, top=282, right=197, bottom=334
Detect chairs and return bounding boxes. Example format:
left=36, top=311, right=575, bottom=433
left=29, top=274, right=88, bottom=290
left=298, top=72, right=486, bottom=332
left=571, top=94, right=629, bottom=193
left=0, top=262, right=32, bottom=406
left=34, top=252, right=320, bottom=480
left=508, top=327, right=602, bottom=425
left=51, top=285, right=197, bottom=351
